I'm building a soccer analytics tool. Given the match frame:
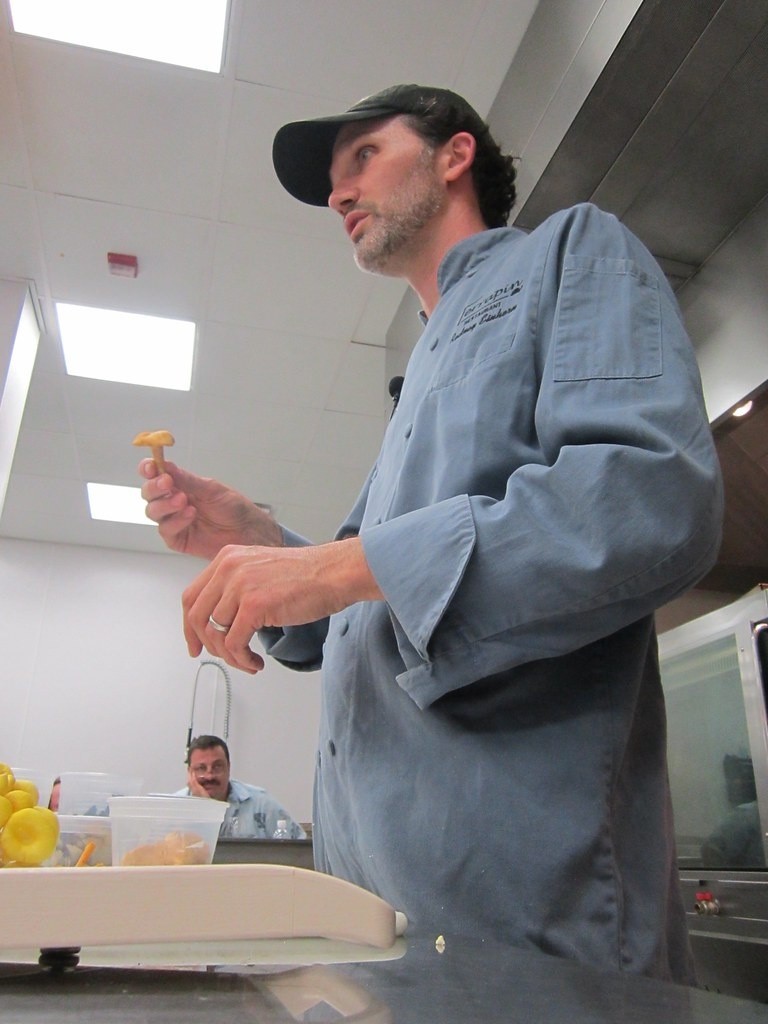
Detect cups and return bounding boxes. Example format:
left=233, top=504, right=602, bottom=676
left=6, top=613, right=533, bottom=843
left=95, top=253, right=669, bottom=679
left=109, top=795, right=230, bottom=867
left=60, top=772, right=141, bottom=814
left=53, top=814, right=112, bottom=866
left=12, top=767, right=55, bottom=812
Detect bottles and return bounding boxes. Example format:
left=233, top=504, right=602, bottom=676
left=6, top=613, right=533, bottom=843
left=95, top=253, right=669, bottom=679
left=272, top=820, right=291, bottom=840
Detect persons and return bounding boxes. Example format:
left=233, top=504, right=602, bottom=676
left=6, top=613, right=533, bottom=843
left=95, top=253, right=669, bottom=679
left=134, top=83, right=725, bottom=991
left=173, top=734, right=307, bottom=840
left=701, top=801, right=766, bottom=868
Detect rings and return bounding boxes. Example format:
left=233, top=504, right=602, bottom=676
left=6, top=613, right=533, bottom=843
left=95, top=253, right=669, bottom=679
left=209, top=614, right=230, bottom=633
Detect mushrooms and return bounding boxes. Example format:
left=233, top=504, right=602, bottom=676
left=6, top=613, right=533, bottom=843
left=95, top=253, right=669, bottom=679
left=132, top=430, right=174, bottom=475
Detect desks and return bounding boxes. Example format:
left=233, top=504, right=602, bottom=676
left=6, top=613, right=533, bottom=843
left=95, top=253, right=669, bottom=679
left=213, top=838, right=314, bottom=871
left=0, top=934, right=768, bottom=1024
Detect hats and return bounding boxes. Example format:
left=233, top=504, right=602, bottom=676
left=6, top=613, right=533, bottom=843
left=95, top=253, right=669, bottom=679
left=273, top=84, right=495, bottom=209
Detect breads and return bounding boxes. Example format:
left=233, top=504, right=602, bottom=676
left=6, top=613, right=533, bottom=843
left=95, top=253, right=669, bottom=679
left=122, top=845, right=163, bottom=865
left=164, top=830, right=210, bottom=865
